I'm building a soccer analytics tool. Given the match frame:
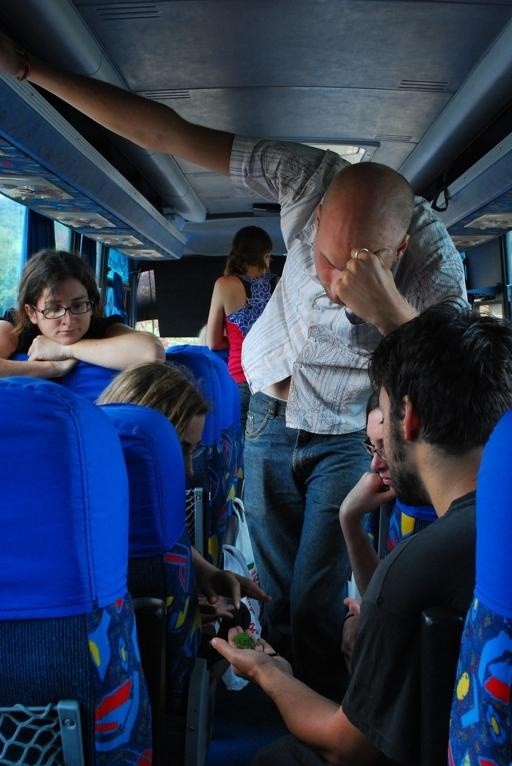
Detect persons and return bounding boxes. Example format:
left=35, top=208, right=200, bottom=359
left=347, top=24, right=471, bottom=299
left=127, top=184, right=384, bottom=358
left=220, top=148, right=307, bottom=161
left=1, top=54, right=512, bottom=766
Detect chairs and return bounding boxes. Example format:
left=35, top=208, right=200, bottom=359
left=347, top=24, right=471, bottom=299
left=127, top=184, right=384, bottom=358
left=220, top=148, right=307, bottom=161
left=373, top=495, right=441, bottom=564
left=0, top=344, right=250, bottom=765
left=441, top=408, right=512, bottom=765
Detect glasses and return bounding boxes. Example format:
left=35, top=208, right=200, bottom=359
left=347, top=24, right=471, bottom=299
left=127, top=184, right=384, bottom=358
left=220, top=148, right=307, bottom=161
left=32, top=300, right=94, bottom=320
left=363, top=441, right=388, bottom=463
left=182, top=443, right=206, bottom=459
